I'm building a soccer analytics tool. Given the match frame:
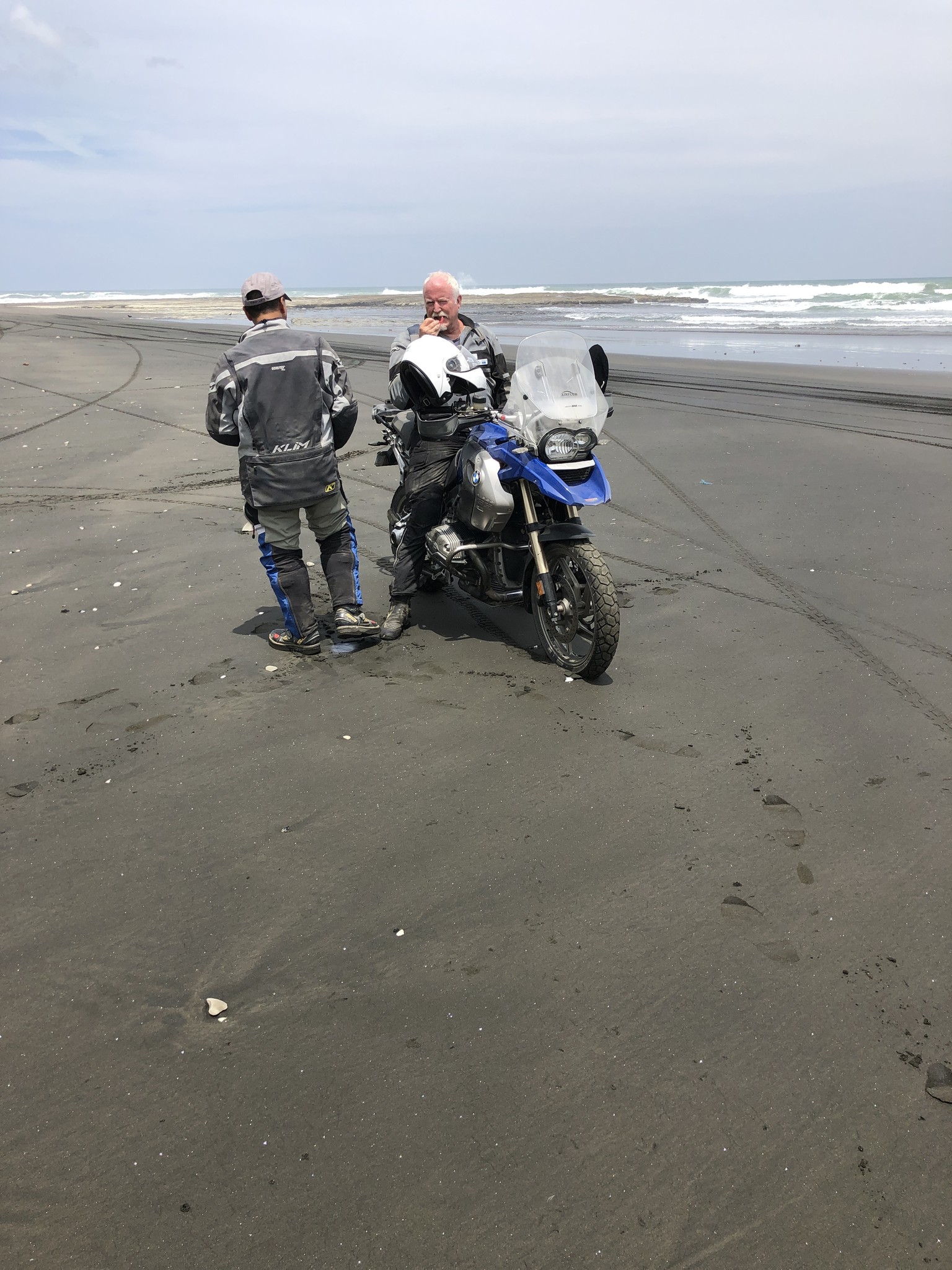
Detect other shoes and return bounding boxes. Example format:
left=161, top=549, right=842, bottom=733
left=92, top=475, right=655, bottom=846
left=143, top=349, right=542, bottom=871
left=334, top=608, right=381, bottom=639
left=379, top=599, right=411, bottom=640
left=268, top=628, right=321, bottom=655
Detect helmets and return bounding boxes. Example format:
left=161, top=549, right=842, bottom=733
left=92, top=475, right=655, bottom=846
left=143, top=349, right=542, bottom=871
left=399, top=335, right=487, bottom=409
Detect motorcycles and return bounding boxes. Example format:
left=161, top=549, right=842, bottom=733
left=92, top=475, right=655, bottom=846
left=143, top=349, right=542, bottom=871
left=367, top=329, right=622, bottom=683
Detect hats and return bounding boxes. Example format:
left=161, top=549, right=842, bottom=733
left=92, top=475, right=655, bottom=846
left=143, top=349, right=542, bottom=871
left=241, top=272, right=292, bottom=306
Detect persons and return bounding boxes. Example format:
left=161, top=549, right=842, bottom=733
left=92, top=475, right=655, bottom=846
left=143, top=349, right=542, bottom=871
left=206, top=273, right=381, bottom=655
left=379, top=272, right=513, bottom=640
